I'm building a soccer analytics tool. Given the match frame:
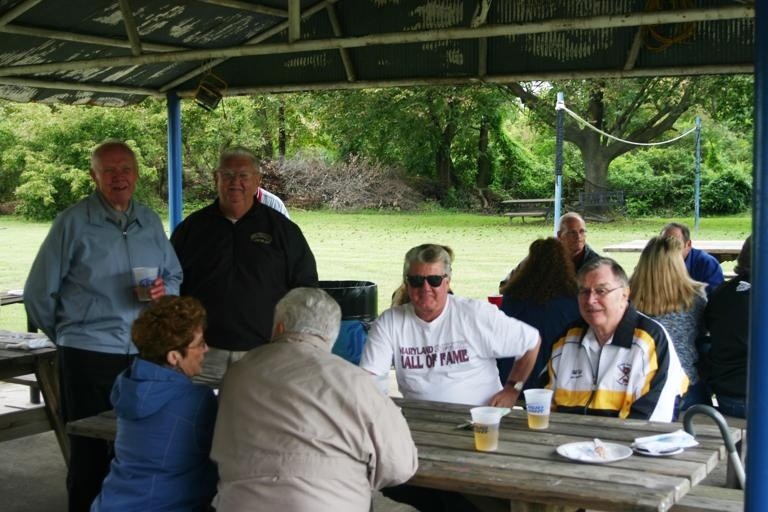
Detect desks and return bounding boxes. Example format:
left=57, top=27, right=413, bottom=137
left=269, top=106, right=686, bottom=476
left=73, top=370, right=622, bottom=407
left=68, top=397, right=747, bottom=511
left=604, top=239, right=746, bottom=265
left=0, top=293, right=40, bottom=404
left=1, top=332, right=70, bottom=474
left=503, top=198, right=566, bottom=211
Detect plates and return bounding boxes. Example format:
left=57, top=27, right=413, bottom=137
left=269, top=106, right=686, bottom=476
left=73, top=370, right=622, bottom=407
left=556, top=441, right=683, bottom=464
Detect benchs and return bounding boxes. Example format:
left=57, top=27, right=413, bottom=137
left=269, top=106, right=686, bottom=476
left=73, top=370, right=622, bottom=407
left=673, top=485, right=745, bottom=510
left=504, top=212, right=550, bottom=226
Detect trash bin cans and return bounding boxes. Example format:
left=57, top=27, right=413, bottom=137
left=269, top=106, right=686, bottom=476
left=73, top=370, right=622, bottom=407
left=319, top=280, right=377, bottom=366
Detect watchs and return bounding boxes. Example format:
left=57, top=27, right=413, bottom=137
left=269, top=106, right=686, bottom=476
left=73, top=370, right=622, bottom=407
left=505, top=378, right=524, bottom=392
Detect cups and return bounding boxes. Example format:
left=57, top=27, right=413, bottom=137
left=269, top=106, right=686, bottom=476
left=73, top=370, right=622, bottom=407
left=524, top=389, right=554, bottom=429
left=132, top=266, right=158, bottom=301
left=470, top=407, right=500, bottom=452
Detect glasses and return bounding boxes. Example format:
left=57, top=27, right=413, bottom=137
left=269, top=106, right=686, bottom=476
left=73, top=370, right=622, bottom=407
left=577, top=285, right=623, bottom=300
left=406, top=271, right=448, bottom=290
left=178, top=337, right=206, bottom=353
left=216, top=170, right=256, bottom=183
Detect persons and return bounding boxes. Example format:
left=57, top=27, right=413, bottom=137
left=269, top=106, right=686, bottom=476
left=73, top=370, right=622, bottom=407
left=358, top=245, right=543, bottom=411
left=85, top=293, right=218, bottom=512
left=626, top=234, right=710, bottom=421
left=660, top=223, right=725, bottom=296
left=168, top=144, right=320, bottom=387
left=389, top=244, right=454, bottom=310
left=212, top=168, right=292, bottom=221
left=498, top=211, right=601, bottom=297
left=701, top=233, right=749, bottom=420
left=207, top=286, right=421, bottom=512
left=513, top=256, right=691, bottom=423
left=493, top=236, right=585, bottom=401
left=23, top=141, right=184, bottom=512
